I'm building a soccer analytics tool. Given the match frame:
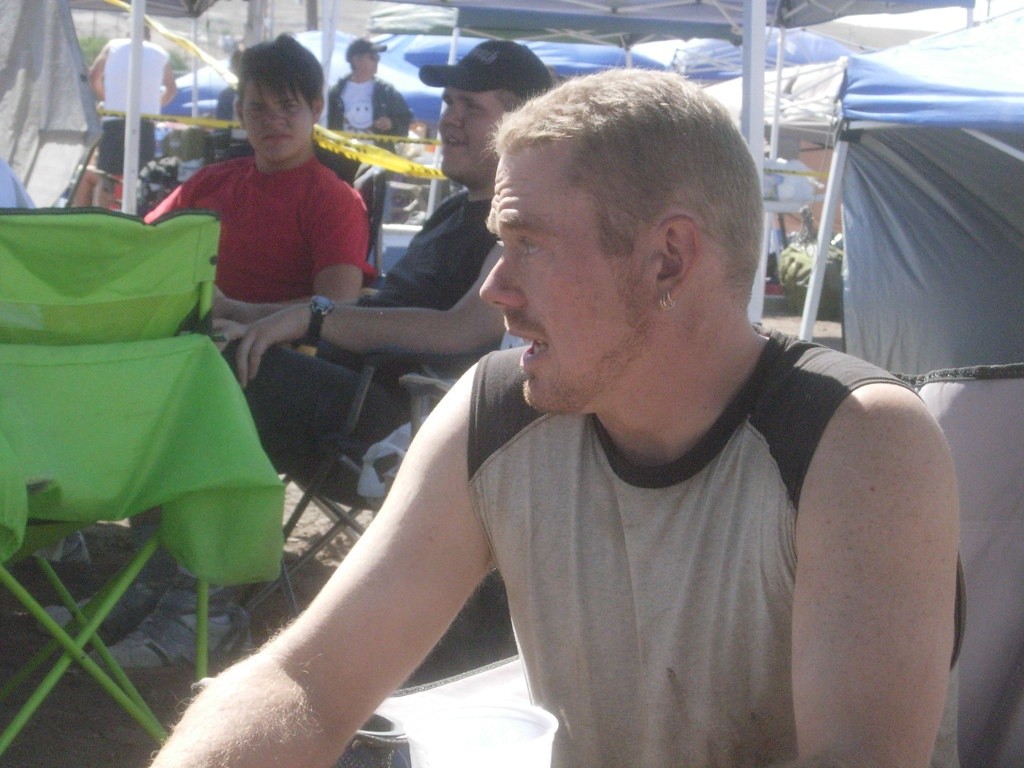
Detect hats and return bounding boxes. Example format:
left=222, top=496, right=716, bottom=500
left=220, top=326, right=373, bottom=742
left=345, top=37, right=387, bottom=63
left=417, top=39, right=553, bottom=98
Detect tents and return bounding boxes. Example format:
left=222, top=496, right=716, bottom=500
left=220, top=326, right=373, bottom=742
left=0, top=1, right=1024, bottom=767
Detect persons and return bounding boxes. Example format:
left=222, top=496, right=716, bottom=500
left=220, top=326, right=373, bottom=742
left=329, top=38, right=410, bottom=259
left=144, top=32, right=370, bottom=357
left=34, top=26, right=556, bottom=686
left=149, top=69, right=969, bottom=768
left=87, top=23, right=177, bottom=210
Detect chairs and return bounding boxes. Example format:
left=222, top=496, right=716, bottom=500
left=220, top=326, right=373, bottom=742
left=239, top=373, right=453, bottom=618
left=63, top=131, right=125, bottom=210
left=225, top=137, right=386, bottom=258
left=1, top=205, right=224, bottom=768
left=338, top=363, right=1024, bottom=768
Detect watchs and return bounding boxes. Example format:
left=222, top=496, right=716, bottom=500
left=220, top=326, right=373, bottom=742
left=304, top=295, right=336, bottom=341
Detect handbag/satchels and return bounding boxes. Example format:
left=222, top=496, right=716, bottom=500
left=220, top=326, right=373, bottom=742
left=780, top=242, right=844, bottom=321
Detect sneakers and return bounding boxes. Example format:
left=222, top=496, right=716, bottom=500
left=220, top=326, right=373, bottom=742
left=79, top=608, right=234, bottom=669
left=37, top=568, right=161, bottom=640
